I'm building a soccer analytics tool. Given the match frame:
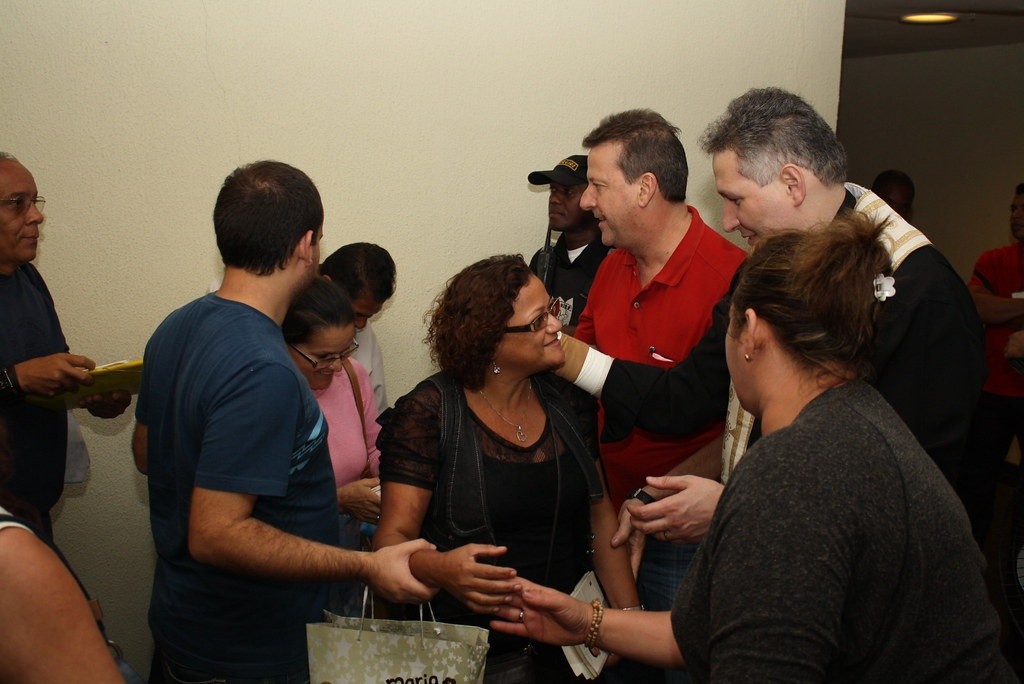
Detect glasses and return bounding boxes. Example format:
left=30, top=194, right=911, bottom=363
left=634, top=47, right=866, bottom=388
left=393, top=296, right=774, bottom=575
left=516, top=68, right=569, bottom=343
left=1, top=195, right=47, bottom=214
left=290, top=338, right=361, bottom=370
left=504, top=298, right=560, bottom=332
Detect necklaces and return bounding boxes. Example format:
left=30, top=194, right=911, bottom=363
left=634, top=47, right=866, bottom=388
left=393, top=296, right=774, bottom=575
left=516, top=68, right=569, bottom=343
left=477, top=378, right=533, bottom=442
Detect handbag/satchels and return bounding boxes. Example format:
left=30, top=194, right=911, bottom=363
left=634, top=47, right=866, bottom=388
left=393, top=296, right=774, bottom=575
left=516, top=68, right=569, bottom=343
left=306, top=583, right=492, bottom=683
left=483, top=644, right=533, bottom=683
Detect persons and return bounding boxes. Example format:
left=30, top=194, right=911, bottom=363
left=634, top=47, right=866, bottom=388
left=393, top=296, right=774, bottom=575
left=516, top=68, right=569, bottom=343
left=0, top=505, right=136, bottom=682
left=132, top=160, right=447, bottom=684
left=287, top=85, right=1024, bottom=684
left=0, top=152, right=131, bottom=541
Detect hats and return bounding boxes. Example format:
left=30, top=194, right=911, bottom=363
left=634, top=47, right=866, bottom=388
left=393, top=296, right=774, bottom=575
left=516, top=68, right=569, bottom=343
left=528, top=155, right=588, bottom=185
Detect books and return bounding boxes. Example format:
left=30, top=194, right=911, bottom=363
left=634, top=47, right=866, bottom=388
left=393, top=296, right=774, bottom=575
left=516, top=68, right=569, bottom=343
left=561, top=572, right=612, bottom=680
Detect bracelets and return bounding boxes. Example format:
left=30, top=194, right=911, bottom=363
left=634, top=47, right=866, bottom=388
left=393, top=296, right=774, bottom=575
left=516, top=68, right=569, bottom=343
left=584, top=599, right=604, bottom=648
left=633, top=488, right=657, bottom=504
left=622, top=605, right=647, bottom=610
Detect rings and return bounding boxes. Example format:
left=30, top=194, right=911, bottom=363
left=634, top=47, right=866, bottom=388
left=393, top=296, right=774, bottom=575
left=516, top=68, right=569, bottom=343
left=376, top=515, right=379, bottom=518
left=519, top=611, right=524, bottom=619
left=664, top=530, right=672, bottom=539
